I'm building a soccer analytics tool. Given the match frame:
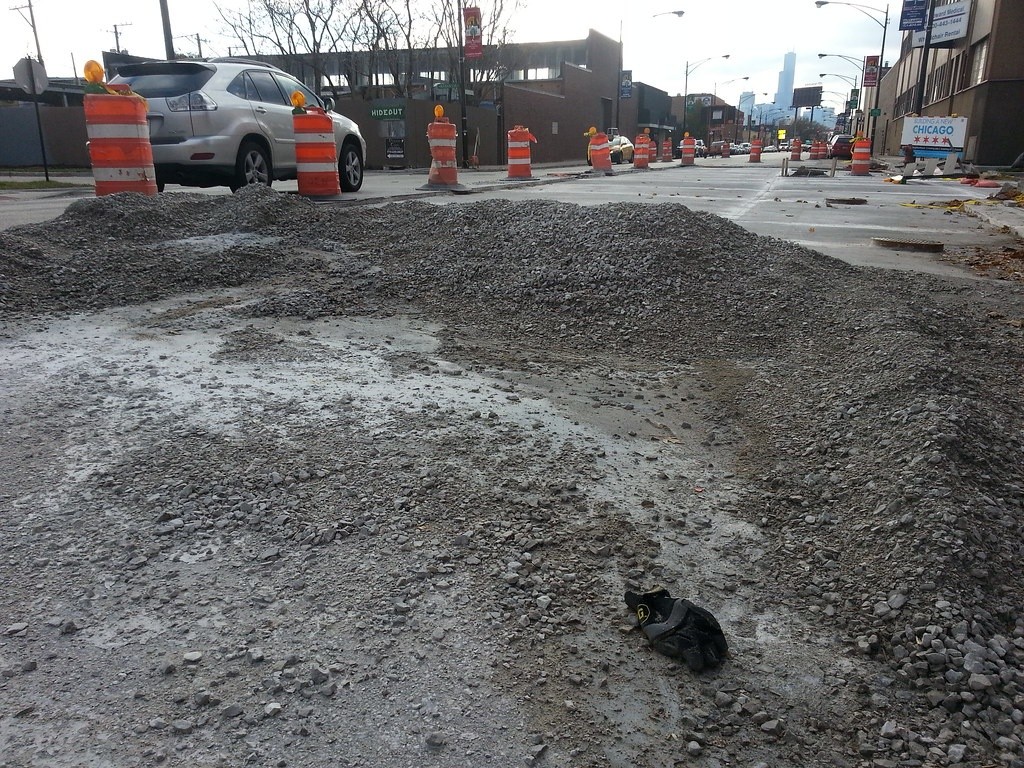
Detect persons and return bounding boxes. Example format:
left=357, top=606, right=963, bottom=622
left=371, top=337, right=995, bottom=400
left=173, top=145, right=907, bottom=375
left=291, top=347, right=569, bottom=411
left=695, top=144, right=717, bottom=159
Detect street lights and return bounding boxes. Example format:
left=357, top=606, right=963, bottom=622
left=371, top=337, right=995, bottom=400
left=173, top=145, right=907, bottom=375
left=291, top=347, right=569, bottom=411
left=748, top=101, right=775, bottom=143
left=819, top=73, right=858, bottom=117
left=615, top=9, right=686, bottom=129
left=713, top=76, right=750, bottom=142
left=815, top=0, right=889, bottom=156
left=818, top=89, right=849, bottom=102
left=682, top=54, right=730, bottom=139
left=759, top=108, right=790, bottom=128
left=819, top=98, right=843, bottom=115
left=817, top=53, right=866, bottom=139
left=734, top=91, right=767, bottom=144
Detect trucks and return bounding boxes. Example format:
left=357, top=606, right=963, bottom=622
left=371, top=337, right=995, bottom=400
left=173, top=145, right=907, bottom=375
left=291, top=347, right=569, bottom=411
left=789, top=138, right=812, bottom=153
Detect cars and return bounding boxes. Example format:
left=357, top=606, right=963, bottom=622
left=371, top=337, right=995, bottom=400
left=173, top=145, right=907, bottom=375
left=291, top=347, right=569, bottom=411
left=826, top=134, right=854, bottom=159
left=586, top=134, right=634, bottom=166
left=708, top=140, right=778, bottom=156
left=676, top=138, right=709, bottom=159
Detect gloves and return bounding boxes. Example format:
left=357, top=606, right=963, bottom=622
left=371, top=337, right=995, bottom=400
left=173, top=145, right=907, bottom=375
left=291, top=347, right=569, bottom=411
left=625, top=591, right=728, bottom=671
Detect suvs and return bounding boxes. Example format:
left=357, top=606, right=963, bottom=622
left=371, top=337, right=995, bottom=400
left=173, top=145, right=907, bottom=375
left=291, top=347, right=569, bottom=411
left=107, top=56, right=368, bottom=195
left=778, top=143, right=789, bottom=152
